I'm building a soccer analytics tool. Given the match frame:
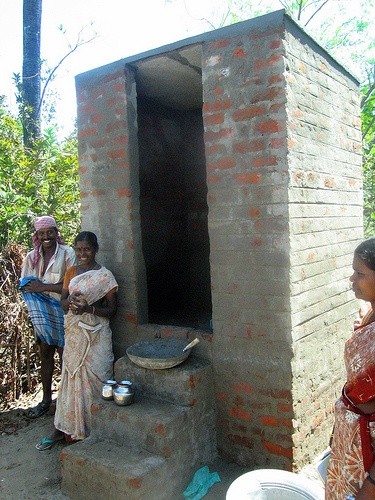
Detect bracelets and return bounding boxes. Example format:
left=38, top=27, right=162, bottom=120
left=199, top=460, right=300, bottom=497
left=67, top=295, right=71, bottom=303
left=93, top=306, right=95, bottom=315
left=366, top=476, right=375, bottom=485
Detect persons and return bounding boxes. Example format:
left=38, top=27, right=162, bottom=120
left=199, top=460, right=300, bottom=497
left=325, top=237, right=375, bottom=500
left=34, top=231, right=119, bottom=449
left=19, top=216, right=78, bottom=418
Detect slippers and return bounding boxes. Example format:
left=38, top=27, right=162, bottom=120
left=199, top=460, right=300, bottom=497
left=27, top=402, right=50, bottom=419
left=36, top=437, right=64, bottom=450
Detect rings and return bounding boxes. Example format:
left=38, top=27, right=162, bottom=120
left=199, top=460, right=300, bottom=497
left=76, top=311, right=77, bottom=313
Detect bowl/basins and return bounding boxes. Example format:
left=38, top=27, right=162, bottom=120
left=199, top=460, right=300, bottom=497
left=225, top=469, right=325, bottom=500
left=126, top=339, right=192, bottom=369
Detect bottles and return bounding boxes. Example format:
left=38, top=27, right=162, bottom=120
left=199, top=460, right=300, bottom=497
left=101, top=380, right=117, bottom=400
left=113, top=380, right=135, bottom=406
